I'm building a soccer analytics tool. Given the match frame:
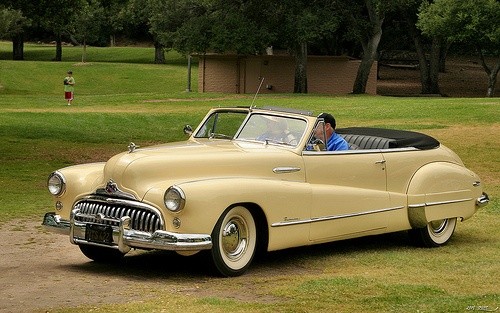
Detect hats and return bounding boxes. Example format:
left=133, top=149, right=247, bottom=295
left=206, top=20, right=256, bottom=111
left=317, top=112, right=336, bottom=130
left=67, top=71, right=72, bottom=74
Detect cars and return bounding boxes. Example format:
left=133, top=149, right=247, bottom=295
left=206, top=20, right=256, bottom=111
left=44, top=104, right=490, bottom=276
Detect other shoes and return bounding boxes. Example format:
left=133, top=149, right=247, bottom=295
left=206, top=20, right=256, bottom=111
left=66, top=103, right=71, bottom=105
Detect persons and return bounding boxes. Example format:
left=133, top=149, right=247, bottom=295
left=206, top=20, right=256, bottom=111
left=307, top=113, right=349, bottom=151
left=62, top=71, right=77, bottom=106
left=255, top=112, right=298, bottom=146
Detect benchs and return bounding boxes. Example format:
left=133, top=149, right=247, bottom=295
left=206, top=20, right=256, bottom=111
left=336, top=134, right=396, bottom=149
